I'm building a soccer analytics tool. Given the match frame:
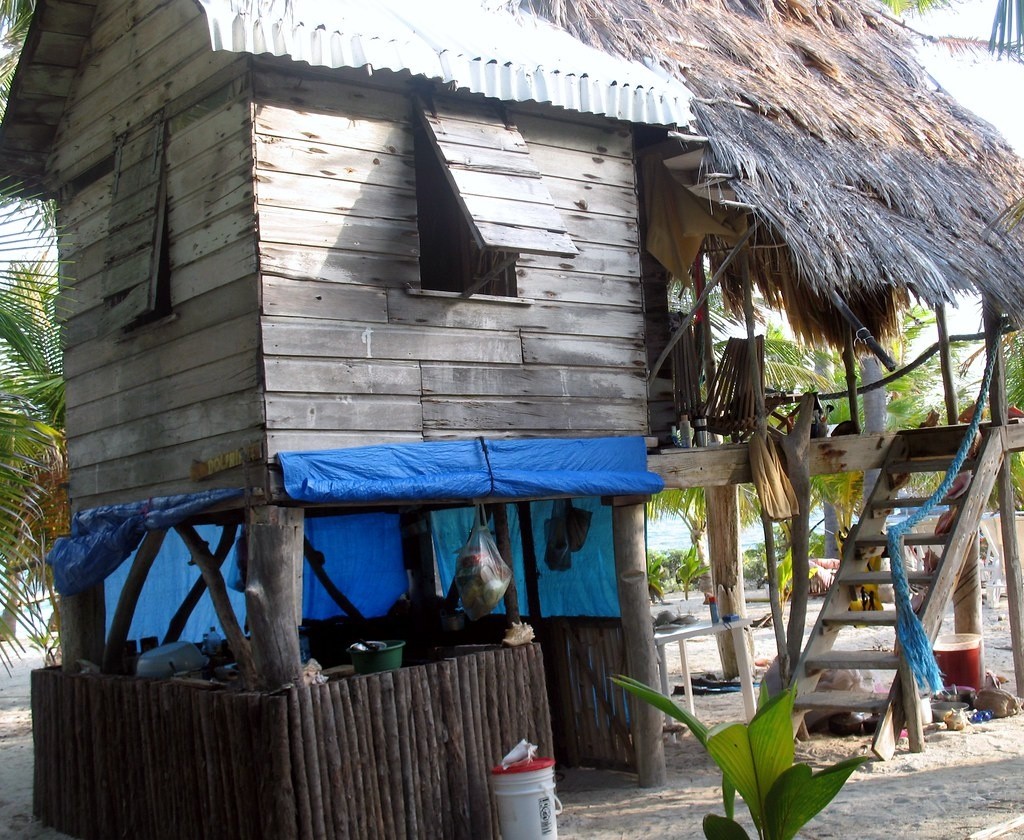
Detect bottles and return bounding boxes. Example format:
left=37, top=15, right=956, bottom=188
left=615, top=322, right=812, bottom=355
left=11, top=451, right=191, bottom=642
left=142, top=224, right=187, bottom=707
left=201, top=633, right=210, bottom=654
left=709, top=597, right=718, bottom=623
left=209, top=627, right=221, bottom=655
left=973, top=709, right=992, bottom=722
left=679, top=415, right=692, bottom=448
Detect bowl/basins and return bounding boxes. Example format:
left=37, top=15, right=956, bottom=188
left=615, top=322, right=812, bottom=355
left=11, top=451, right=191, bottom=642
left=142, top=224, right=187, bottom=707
left=346, top=639, right=407, bottom=675
left=932, top=633, right=982, bottom=651
left=135, top=641, right=209, bottom=681
left=929, top=702, right=970, bottom=722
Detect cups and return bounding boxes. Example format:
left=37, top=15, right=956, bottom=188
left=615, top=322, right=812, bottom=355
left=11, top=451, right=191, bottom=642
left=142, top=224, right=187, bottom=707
left=694, top=415, right=708, bottom=447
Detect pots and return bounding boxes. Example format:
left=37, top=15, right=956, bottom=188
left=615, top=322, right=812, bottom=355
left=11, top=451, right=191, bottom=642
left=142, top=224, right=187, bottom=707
left=863, top=713, right=881, bottom=734
left=828, top=712, right=864, bottom=736
left=938, top=689, right=976, bottom=710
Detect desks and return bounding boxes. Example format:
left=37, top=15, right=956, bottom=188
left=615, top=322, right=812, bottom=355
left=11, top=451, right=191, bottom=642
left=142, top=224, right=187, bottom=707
left=654, top=618, right=757, bottom=745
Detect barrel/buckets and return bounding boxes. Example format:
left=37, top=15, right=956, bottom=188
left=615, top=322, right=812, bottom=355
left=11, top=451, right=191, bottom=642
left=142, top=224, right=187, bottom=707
left=932, top=646, right=980, bottom=696
left=491, top=757, right=562, bottom=840
